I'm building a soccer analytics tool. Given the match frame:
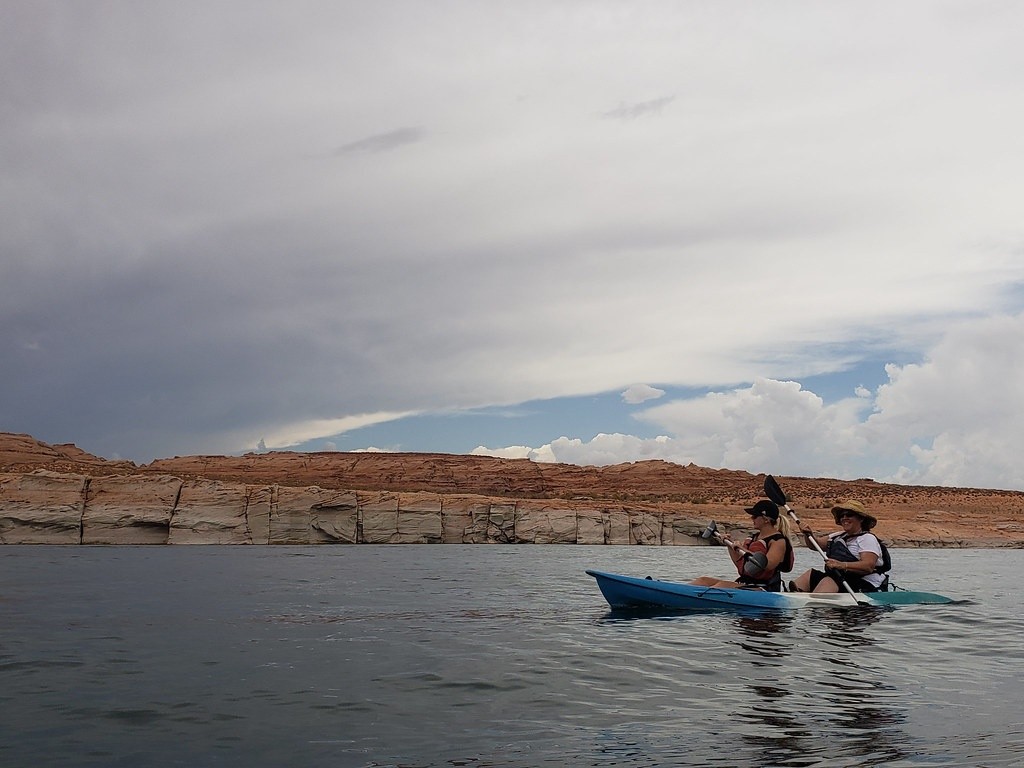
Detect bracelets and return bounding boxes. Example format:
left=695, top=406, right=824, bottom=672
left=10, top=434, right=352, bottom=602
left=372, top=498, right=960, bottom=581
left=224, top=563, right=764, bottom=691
left=845, top=562, right=848, bottom=570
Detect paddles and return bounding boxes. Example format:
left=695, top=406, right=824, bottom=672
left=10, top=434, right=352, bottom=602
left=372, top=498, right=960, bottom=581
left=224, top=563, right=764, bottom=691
left=762, top=473, right=872, bottom=607
left=701, top=518, right=769, bottom=574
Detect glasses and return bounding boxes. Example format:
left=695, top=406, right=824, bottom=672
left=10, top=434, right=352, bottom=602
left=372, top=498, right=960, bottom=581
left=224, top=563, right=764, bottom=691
left=837, top=512, right=856, bottom=518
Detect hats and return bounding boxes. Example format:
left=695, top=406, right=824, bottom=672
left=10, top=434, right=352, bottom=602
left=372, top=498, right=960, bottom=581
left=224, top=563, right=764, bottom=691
left=831, top=500, right=877, bottom=529
left=744, top=500, right=778, bottom=519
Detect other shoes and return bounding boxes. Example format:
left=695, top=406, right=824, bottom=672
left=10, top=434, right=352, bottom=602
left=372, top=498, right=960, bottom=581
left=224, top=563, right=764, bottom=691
left=789, top=581, right=800, bottom=592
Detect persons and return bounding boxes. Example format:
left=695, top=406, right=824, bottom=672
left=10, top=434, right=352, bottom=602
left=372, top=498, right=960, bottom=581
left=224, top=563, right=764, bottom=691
left=645, top=500, right=786, bottom=592
left=790, top=501, right=886, bottom=593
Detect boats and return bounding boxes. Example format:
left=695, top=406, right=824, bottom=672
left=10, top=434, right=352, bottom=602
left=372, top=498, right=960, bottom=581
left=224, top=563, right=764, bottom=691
left=584, top=569, right=954, bottom=612
